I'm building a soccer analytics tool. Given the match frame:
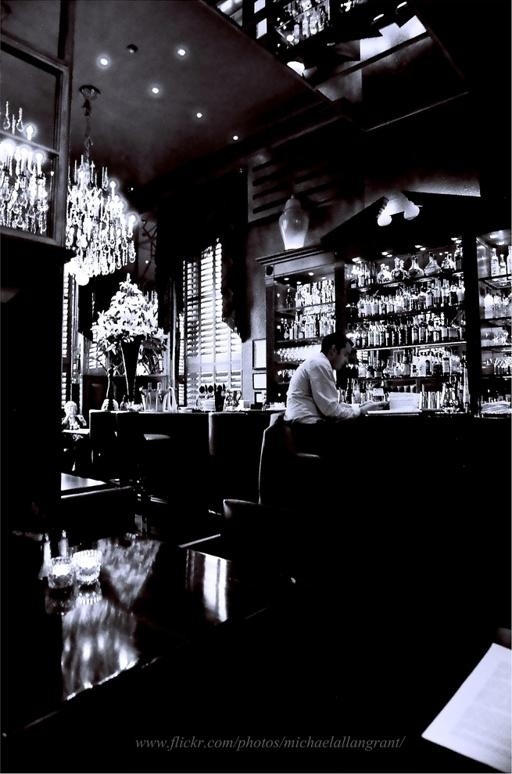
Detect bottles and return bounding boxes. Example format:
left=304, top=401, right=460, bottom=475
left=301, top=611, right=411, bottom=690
left=36, top=531, right=68, bottom=589
left=139, top=381, right=164, bottom=413
left=271, top=238, right=512, bottom=413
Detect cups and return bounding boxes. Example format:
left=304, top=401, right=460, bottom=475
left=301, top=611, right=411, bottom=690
left=45, top=550, right=100, bottom=590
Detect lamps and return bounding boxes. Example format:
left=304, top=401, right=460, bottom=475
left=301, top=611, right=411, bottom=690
left=67, top=83, right=141, bottom=286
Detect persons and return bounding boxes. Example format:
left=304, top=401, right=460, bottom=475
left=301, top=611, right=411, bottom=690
left=282, top=331, right=385, bottom=455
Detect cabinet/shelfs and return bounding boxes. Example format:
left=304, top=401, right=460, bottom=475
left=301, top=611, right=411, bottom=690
left=254, top=189, right=511, bottom=418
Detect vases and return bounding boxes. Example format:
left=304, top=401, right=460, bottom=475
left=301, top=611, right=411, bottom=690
left=118, top=336, right=147, bottom=412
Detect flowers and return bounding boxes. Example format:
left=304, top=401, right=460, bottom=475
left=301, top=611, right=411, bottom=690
left=93, top=274, right=167, bottom=354
